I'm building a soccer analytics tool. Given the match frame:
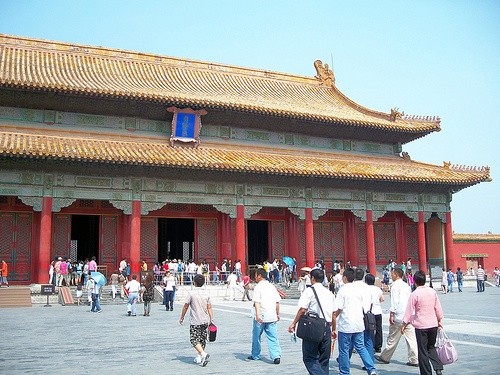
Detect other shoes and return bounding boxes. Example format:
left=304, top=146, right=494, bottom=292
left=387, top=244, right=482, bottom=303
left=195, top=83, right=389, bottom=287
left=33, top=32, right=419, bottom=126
left=247, top=356, right=261, bottom=360
left=362, top=367, right=366, bottom=370
left=376, top=348, right=381, bottom=352
left=436, top=370, right=443, bottom=375
left=374, top=355, right=389, bottom=364
left=97, top=309, right=103, bottom=314
left=370, top=372, right=377, bottom=375
left=273, top=358, right=280, bottom=364
left=407, top=362, right=419, bottom=367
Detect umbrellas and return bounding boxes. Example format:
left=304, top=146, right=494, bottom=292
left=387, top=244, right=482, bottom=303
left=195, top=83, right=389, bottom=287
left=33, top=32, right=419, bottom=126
left=91, top=271, right=107, bottom=286
left=282, top=256, right=295, bottom=266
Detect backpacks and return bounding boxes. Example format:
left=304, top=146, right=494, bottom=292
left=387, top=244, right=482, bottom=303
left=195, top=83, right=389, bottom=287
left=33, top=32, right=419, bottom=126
left=143, top=285, right=153, bottom=302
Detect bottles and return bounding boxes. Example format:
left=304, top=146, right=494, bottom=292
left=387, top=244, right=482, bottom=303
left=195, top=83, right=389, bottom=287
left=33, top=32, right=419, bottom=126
left=290, top=329, right=297, bottom=344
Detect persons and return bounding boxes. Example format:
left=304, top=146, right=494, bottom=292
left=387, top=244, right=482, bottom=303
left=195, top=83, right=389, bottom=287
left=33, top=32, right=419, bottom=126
left=374, top=268, right=419, bottom=367
left=48, top=256, right=97, bottom=287
left=283, top=264, right=293, bottom=289
left=74, top=277, right=103, bottom=313
left=292, top=257, right=298, bottom=283
left=249, top=258, right=283, bottom=284
left=141, top=258, right=252, bottom=312
left=124, top=274, right=155, bottom=317
left=179, top=273, right=213, bottom=367
left=401, top=270, right=444, bottom=375
left=298, top=259, right=371, bottom=297
left=380, top=257, right=417, bottom=292
left=109, top=258, right=130, bottom=299
left=247, top=268, right=282, bottom=364
left=288, top=270, right=336, bottom=375
left=0, top=260, right=9, bottom=287
left=441, top=264, right=500, bottom=294
left=329, top=269, right=385, bottom=375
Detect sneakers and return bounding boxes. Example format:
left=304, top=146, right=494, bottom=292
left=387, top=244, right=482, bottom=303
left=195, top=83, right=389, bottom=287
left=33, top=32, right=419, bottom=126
left=200, top=352, right=210, bottom=367
left=193, top=357, right=202, bottom=364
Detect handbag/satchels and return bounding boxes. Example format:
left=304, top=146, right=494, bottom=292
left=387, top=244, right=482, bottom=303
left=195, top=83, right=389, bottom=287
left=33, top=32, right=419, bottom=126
left=296, top=312, right=327, bottom=343
left=208, top=323, right=218, bottom=343
left=364, top=309, right=377, bottom=331
left=435, top=327, right=459, bottom=366
